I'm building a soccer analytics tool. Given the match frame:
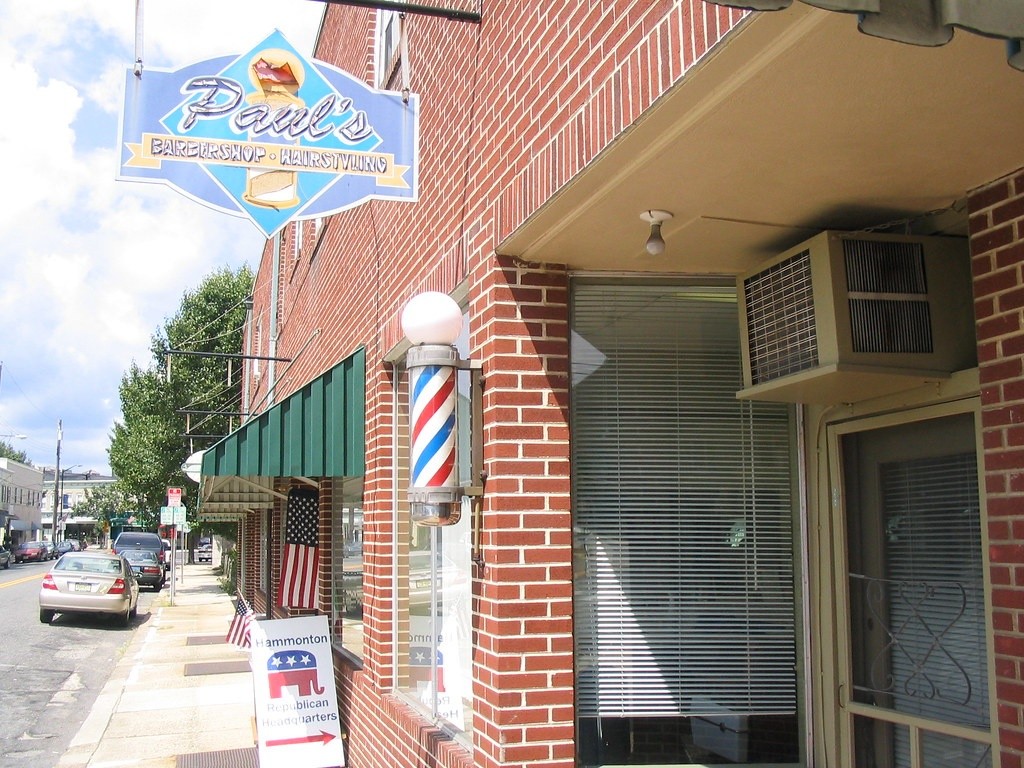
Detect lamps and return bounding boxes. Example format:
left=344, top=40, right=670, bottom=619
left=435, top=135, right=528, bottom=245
left=400, top=289, right=465, bottom=349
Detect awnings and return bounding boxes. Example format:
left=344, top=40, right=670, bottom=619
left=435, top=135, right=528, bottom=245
left=10, top=520, right=31, bottom=530
left=196, top=347, right=366, bottom=522
left=32, top=522, right=42, bottom=530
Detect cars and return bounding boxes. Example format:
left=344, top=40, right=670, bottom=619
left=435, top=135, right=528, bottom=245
left=107, top=549, right=171, bottom=592
left=0, top=545, right=11, bottom=569
left=39, top=552, right=144, bottom=628
left=409, top=551, right=471, bottom=609
left=160, top=538, right=172, bottom=570
left=198, top=537, right=212, bottom=562
left=15, top=539, right=81, bottom=564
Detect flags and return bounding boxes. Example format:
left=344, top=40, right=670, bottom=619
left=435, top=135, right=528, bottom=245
left=278, top=488, right=319, bottom=609
left=225, top=598, right=252, bottom=648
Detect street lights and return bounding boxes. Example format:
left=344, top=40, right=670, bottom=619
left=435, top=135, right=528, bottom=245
left=60, top=464, right=83, bottom=546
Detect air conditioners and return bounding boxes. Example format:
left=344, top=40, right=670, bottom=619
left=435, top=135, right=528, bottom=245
left=736, top=229, right=978, bottom=386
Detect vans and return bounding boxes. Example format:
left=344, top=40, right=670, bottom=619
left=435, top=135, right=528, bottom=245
left=110, top=532, right=167, bottom=572
